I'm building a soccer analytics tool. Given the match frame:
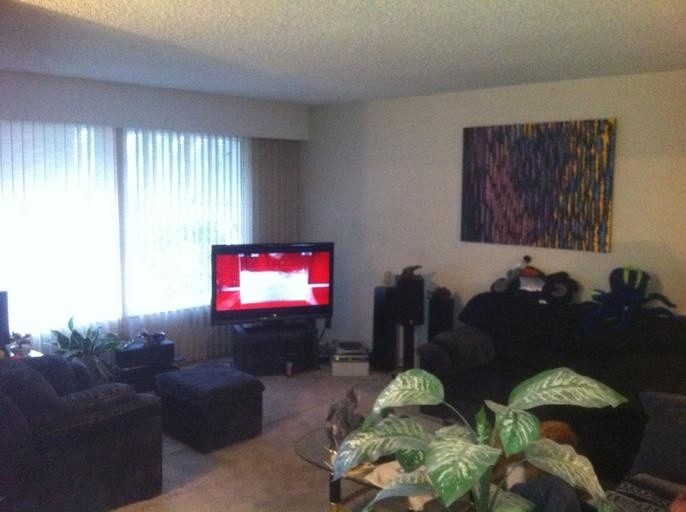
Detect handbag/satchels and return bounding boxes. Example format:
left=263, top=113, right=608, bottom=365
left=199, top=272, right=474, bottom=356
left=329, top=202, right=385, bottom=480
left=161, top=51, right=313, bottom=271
left=326, top=384, right=365, bottom=450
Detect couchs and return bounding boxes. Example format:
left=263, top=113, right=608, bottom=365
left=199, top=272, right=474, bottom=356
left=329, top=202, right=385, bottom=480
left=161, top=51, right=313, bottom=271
left=1, top=358, right=161, bottom=511
left=153, top=363, right=264, bottom=453
left=423, top=292, right=686, bottom=483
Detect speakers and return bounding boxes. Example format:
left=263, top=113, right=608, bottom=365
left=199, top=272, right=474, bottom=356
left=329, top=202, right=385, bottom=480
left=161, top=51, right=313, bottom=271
left=427, top=298, right=454, bottom=342
left=372, top=286, right=398, bottom=373
left=395, top=274, right=424, bottom=326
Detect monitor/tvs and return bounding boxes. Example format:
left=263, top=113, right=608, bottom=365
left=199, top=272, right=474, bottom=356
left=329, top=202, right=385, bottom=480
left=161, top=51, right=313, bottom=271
left=211, top=242, right=334, bottom=333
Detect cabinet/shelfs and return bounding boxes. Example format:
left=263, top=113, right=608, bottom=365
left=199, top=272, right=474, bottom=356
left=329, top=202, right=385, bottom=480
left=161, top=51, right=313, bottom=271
left=116, top=338, right=175, bottom=391
left=231, top=320, right=315, bottom=371
